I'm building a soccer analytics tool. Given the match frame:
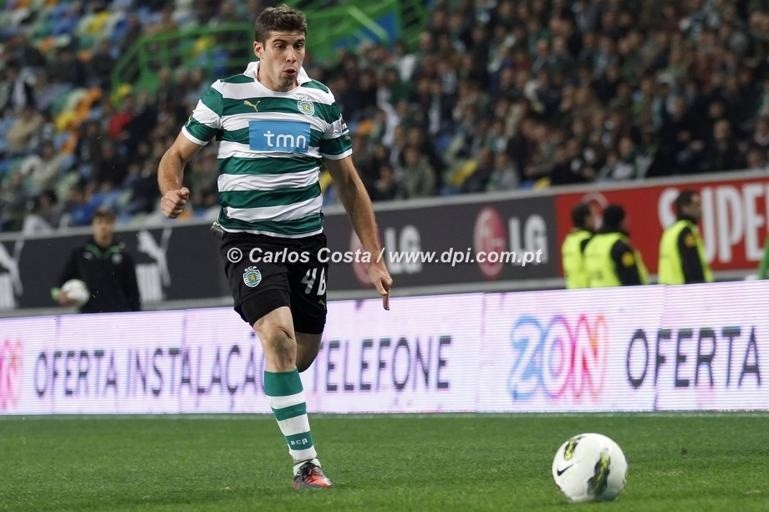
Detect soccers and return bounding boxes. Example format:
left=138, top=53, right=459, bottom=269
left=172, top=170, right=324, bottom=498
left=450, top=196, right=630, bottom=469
left=554, top=431, right=629, bottom=503
left=59, top=280, right=89, bottom=310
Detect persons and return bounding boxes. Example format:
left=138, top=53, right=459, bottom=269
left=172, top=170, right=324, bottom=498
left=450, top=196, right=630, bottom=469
left=51, top=208, right=142, bottom=314
left=583, top=205, right=650, bottom=288
left=561, top=204, right=596, bottom=289
left=158, top=4, right=393, bottom=489
left=659, top=190, right=714, bottom=284
left=0, top=0, right=766, bottom=232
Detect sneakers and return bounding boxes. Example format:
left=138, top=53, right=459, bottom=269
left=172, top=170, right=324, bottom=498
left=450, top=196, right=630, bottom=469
left=292, top=458, right=333, bottom=491
